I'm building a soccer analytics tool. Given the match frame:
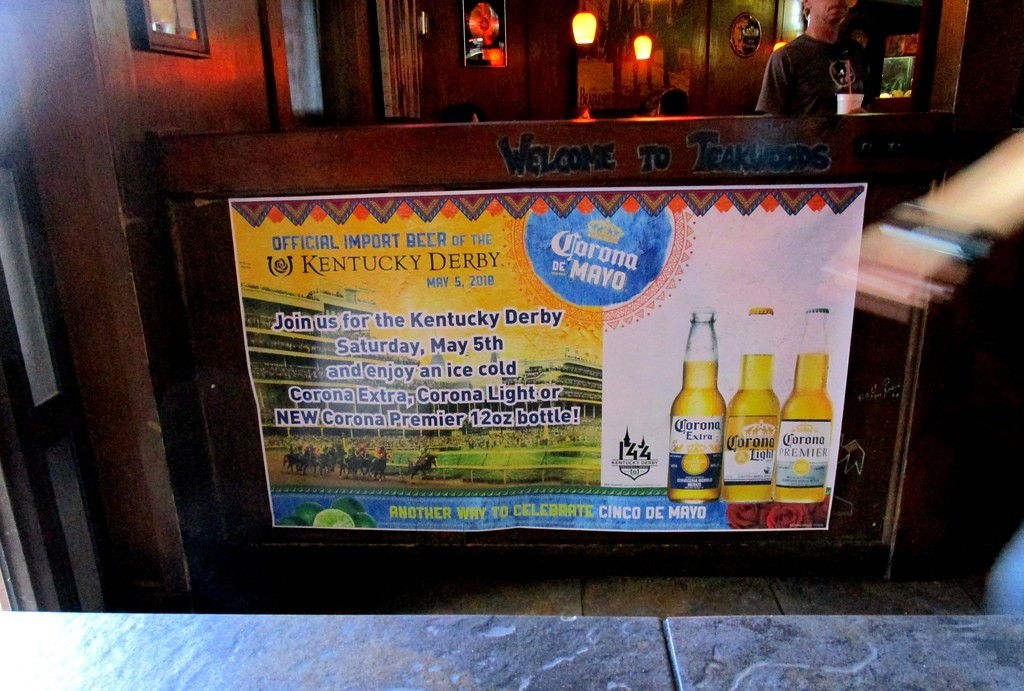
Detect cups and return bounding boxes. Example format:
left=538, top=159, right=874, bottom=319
left=836, top=93, right=864, bottom=115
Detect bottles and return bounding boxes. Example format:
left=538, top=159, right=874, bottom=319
left=722, top=307, right=781, bottom=509
left=770, top=307, right=834, bottom=504
left=666, top=309, right=727, bottom=505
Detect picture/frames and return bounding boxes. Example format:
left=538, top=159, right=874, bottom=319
left=730, top=12, right=762, bottom=57
left=129, top=0, right=211, bottom=59
left=461, top=0, right=508, bottom=68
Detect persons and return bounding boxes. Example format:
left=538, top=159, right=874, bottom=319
left=827, top=127, right=1024, bottom=616
left=755, top=0, right=874, bottom=114
left=656, top=87, right=689, bottom=118
left=441, top=103, right=480, bottom=123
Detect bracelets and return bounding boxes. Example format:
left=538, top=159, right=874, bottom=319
left=882, top=205, right=997, bottom=261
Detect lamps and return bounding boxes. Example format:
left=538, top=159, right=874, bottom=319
left=572, top=0, right=653, bottom=60
